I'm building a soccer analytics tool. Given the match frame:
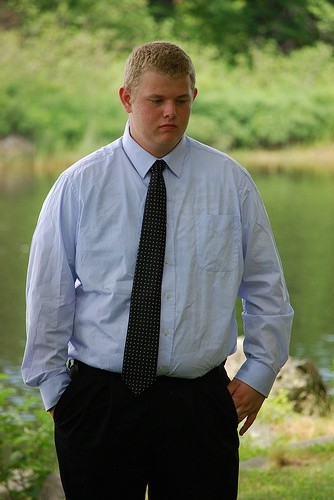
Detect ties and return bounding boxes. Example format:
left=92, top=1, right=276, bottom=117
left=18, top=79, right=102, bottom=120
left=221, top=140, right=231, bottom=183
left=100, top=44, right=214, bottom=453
left=123, top=160, right=168, bottom=395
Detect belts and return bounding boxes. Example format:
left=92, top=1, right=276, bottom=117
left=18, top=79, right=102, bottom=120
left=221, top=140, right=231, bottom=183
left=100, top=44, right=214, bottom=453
left=73, top=359, right=223, bottom=388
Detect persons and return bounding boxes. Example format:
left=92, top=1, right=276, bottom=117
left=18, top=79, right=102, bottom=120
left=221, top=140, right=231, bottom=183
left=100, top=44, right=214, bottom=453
left=21, top=41, right=295, bottom=500
